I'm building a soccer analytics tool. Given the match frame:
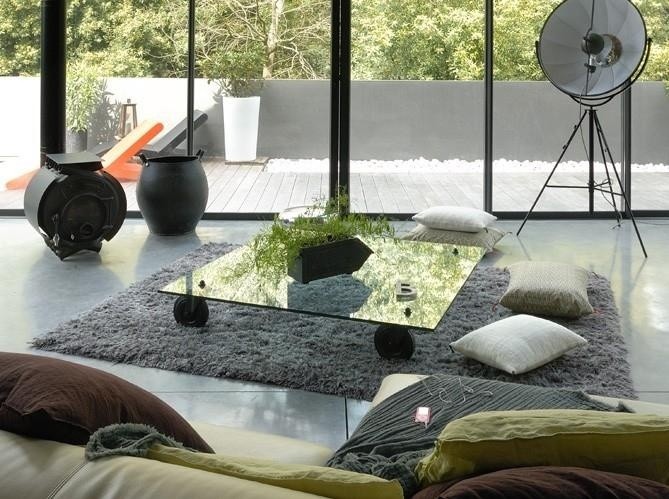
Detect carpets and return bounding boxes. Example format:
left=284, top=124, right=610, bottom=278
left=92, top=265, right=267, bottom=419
left=29, top=239, right=642, bottom=409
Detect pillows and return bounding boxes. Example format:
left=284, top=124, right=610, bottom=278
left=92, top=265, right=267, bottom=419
left=410, top=204, right=497, bottom=235
left=412, top=463, right=669, bottom=499
left=400, top=222, right=514, bottom=255
left=409, top=405, right=669, bottom=489
left=137, top=440, right=407, bottom=497
left=446, top=313, right=589, bottom=378
left=0, top=348, right=216, bottom=454
left=496, top=258, right=596, bottom=320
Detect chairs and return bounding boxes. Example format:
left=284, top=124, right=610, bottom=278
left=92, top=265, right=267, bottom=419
left=85, top=108, right=208, bottom=161
left=4, top=118, right=164, bottom=193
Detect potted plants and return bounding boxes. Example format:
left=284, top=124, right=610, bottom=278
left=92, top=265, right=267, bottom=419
left=198, top=39, right=272, bottom=163
left=196, top=174, right=402, bottom=300
left=63, top=56, right=112, bottom=155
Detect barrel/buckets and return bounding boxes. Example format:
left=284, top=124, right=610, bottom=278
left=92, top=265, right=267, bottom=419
left=277, top=205, right=340, bottom=229
left=136, top=148, right=209, bottom=235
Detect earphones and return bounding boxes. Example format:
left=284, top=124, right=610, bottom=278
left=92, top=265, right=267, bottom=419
left=484, top=391, right=494, bottom=397
left=464, top=386, right=473, bottom=394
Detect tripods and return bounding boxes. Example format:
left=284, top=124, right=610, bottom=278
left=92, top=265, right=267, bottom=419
left=517, top=108, right=647, bottom=259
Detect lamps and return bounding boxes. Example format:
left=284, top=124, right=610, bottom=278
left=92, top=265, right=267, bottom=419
left=516, top=0, right=653, bottom=259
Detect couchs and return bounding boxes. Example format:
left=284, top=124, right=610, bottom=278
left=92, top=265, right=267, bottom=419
left=0, top=373, right=669, bottom=498
